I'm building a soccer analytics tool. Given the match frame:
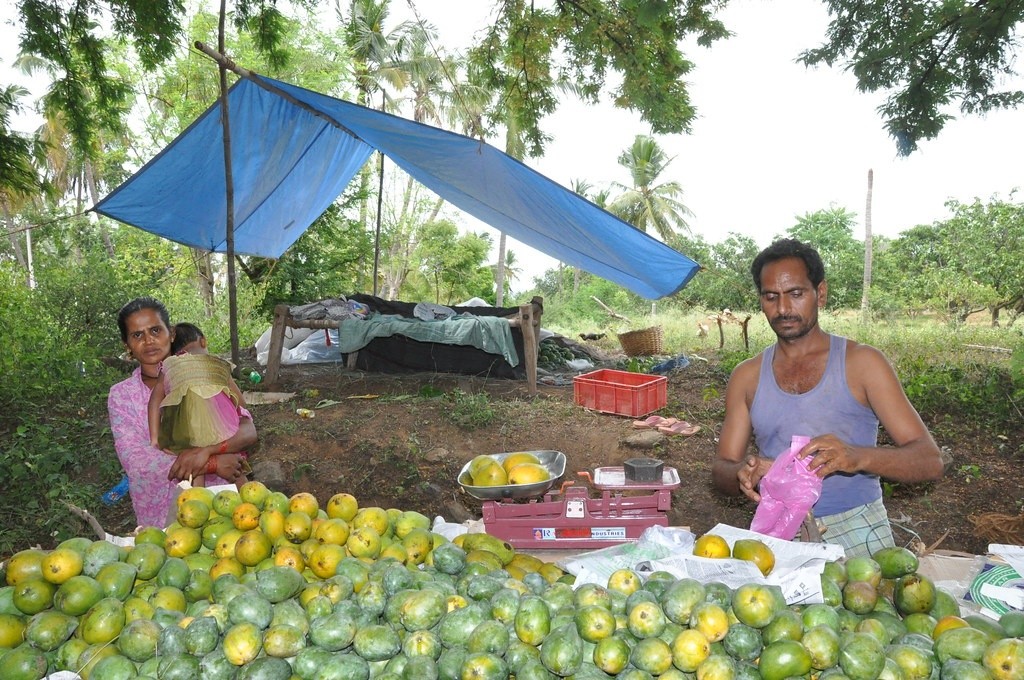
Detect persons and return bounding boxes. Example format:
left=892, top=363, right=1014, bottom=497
left=712, top=237, right=943, bottom=560
left=107, top=297, right=259, bottom=526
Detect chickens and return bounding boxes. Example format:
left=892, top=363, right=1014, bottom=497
left=696, top=322, right=709, bottom=340
left=578, top=332, right=606, bottom=341
left=566, top=359, right=594, bottom=375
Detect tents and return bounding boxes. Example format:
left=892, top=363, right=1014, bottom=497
left=92, top=0, right=705, bottom=375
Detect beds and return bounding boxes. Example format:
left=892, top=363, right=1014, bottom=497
left=263, top=293, right=542, bottom=396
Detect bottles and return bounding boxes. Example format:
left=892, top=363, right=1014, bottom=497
left=102, top=476, right=129, bottom=507
left=250, top=371, right=261, bottom=384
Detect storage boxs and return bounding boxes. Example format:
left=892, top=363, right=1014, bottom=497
left=572, top=369, right=668, bottom=417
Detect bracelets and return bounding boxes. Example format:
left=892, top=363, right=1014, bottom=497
left=220, top=441, right=227, bottom=453
left=207, top=454, right=217, bottom=474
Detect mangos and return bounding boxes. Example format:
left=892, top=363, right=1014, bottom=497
left=0, top=452, right=1024, bottom=680
